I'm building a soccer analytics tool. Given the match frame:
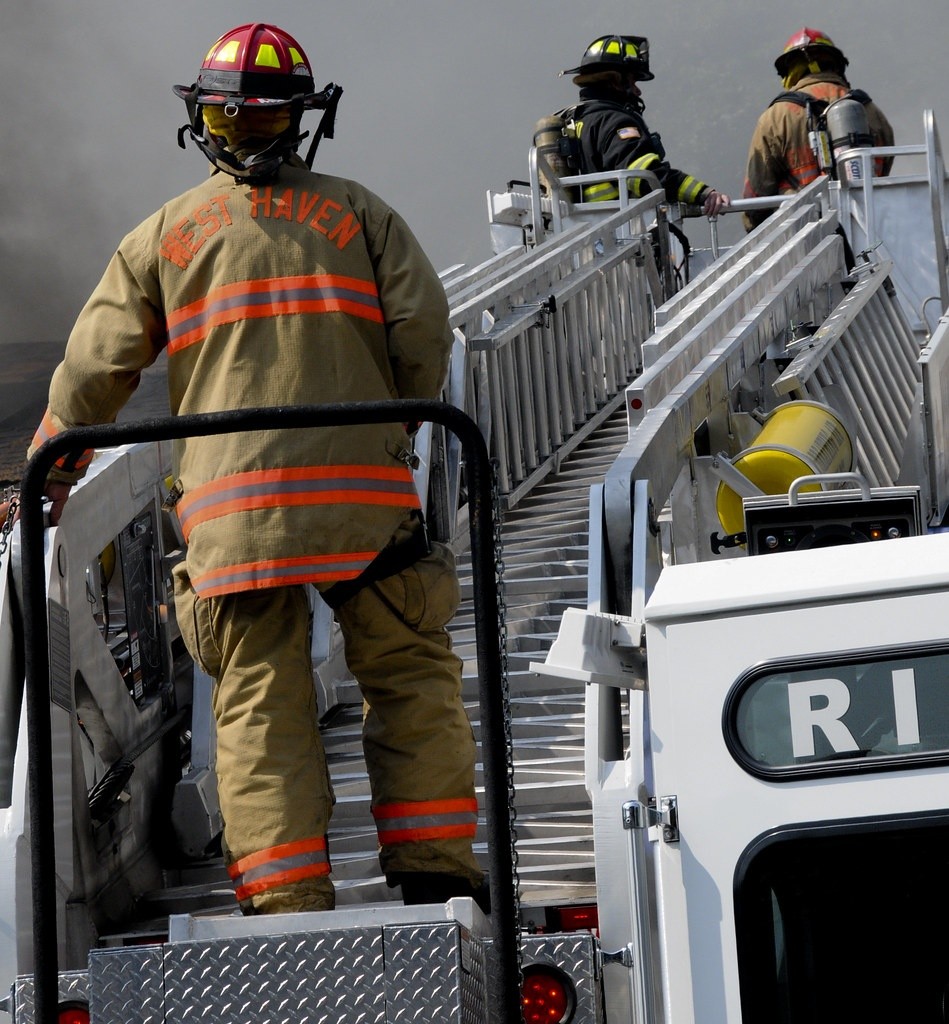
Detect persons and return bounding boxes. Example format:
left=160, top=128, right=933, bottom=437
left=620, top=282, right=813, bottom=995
left=12, top=22, right=490, bottom=917
left=742, top=28, right=895, bottom=276
left=560, top=35, right=729, bottom=280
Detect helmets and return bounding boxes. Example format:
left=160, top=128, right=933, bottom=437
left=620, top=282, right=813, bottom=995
left=774, top=27, right=849, bottom=79
left=557, top=34, right=655, bottom=81
left=171, top=22, right=344, bottom=117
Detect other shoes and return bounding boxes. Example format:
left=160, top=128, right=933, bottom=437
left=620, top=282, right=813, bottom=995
left=399, top=868, right=524, bottom=915
